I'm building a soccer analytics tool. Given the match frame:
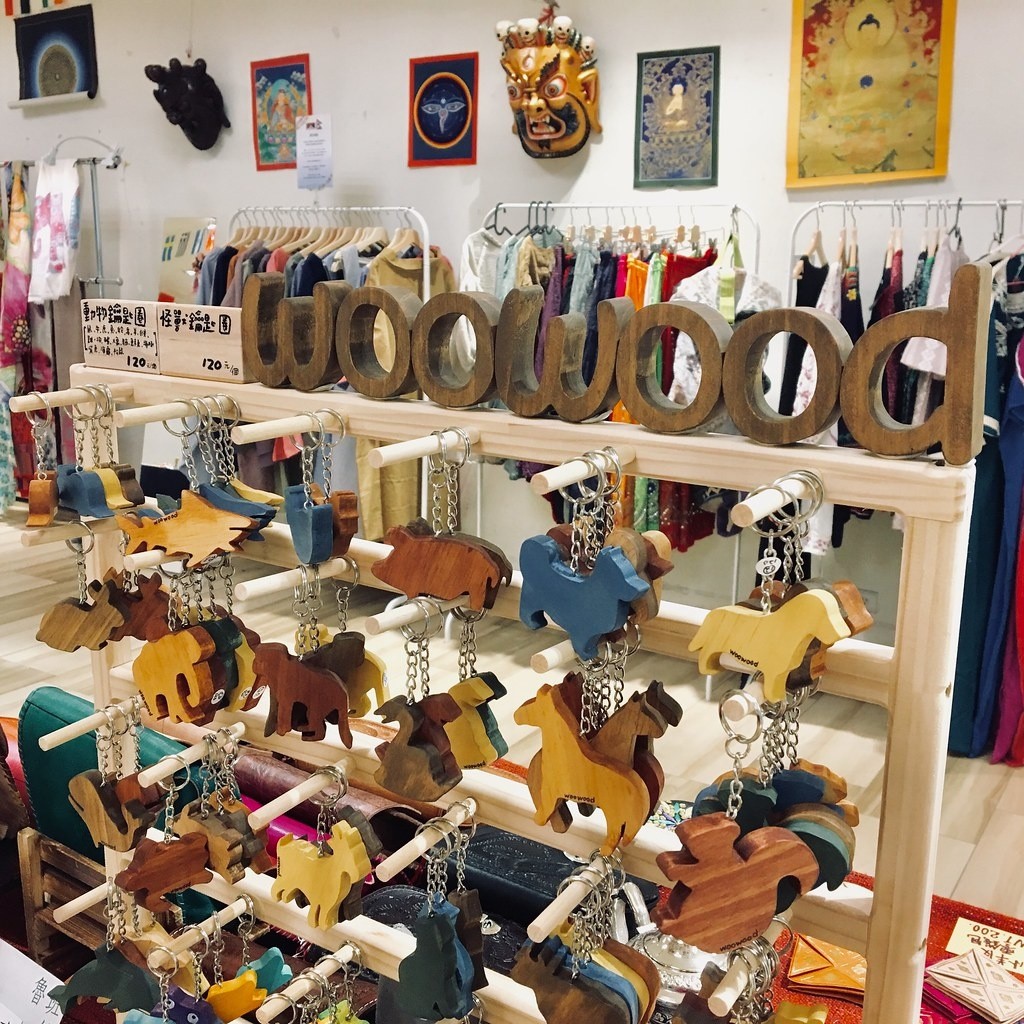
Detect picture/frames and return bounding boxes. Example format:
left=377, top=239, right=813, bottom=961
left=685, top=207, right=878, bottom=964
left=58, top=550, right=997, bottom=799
left=249, top=53, right=312, bottom=171
left=407, top=51, right=479, bottom=168
left=785, top=0, right=959, bottom=188
left=633, top=46, right=721, bottom=185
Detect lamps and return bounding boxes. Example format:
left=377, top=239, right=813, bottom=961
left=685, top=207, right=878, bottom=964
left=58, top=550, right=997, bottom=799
left=40, top=135, right=122, bottom=166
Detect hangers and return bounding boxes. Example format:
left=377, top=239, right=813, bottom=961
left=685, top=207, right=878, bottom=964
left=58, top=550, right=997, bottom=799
left=482, top=200, right=741, bottom=257
left=789, top=196, right=1024, bottom=280
left=218, top=204, right=436, bottom=258
